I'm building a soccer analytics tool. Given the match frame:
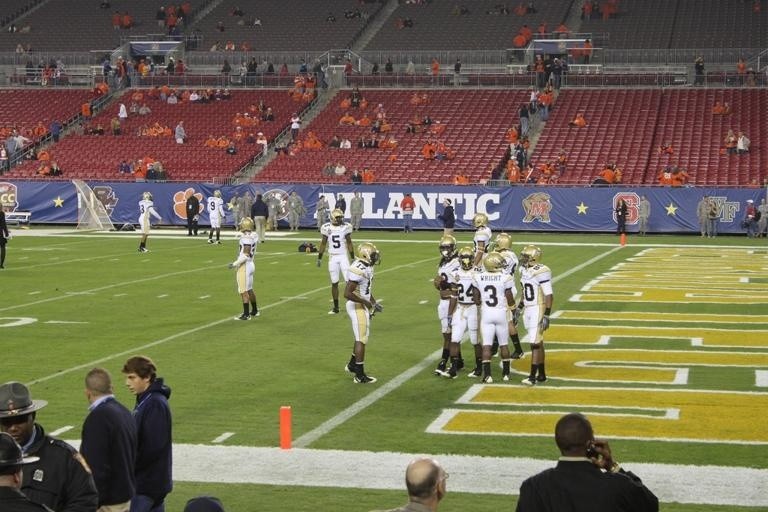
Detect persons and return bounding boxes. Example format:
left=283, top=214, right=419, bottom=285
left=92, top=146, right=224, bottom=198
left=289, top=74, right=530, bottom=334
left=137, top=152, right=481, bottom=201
left=317, top=209, right=355, bottom=315
left=340, top=241, right=383, bottom=385
left=1, top=355, right=230, bottom=512
left=1, top=1, right=768, bottom=262
left=437, top=198, right=554, bottom=388
left=229, top=217, right=260, bottom=320
left=517, top=409, right=662, bottom=510
left=371, top=458, right=455, bottom=512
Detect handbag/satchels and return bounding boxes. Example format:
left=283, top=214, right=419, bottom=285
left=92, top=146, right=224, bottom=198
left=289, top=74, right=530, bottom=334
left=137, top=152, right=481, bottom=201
left=299, top=242, right=317, bottom=252
left=752, top=211, right=761, bottom=221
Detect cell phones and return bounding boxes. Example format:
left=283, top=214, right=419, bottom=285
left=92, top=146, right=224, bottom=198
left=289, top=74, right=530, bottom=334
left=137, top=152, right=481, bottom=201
left=585, top=443, right=597, bottom=460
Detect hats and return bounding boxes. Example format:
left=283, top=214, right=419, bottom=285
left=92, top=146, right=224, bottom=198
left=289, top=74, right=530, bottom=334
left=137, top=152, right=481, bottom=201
left=0, top=379, right=49, bottom=418
left=746, top=200, right=753, bottom=203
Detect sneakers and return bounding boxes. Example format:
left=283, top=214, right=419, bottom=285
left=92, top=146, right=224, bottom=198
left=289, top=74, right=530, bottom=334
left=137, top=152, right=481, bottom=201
left=137, top=247, right=150, bottom=253
left=249, top=310, right=262, bottom=316
left=352, top=374, right=377, bottom=384
left=433, top=349, right=551, bottom=386
left=343, top=361, right=356, bottom=374
left=233, top=312, right=252, bottom=321
left=208, top=239, right=214, bottom=244
left=326, top=307, right=340, bottom=315
left=215, top=240, right=222, bottom=245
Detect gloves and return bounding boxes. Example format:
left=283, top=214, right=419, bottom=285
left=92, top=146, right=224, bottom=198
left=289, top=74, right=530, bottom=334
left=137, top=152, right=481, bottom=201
left=511, top=309, right=521, bottom=324
left=316, top=259, right=322, bottom=267
left=227, top=263, right=233, bottom=270
left=538, top=316, right=550, bottom=332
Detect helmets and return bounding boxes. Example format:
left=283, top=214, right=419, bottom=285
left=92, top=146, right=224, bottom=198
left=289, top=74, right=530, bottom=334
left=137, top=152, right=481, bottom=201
left=237, top=216, right=256, bottom=233
left=213, top=190, right=223, bottom=198
left=472, top=212, right=489, bottom=229
left=434, top=230, right=543, bottom=273
left=141, top=192, right=154, bottom=201
left=329, top=208, right=345, bottom=225
left=354, top=241, right=381, bottom=267
